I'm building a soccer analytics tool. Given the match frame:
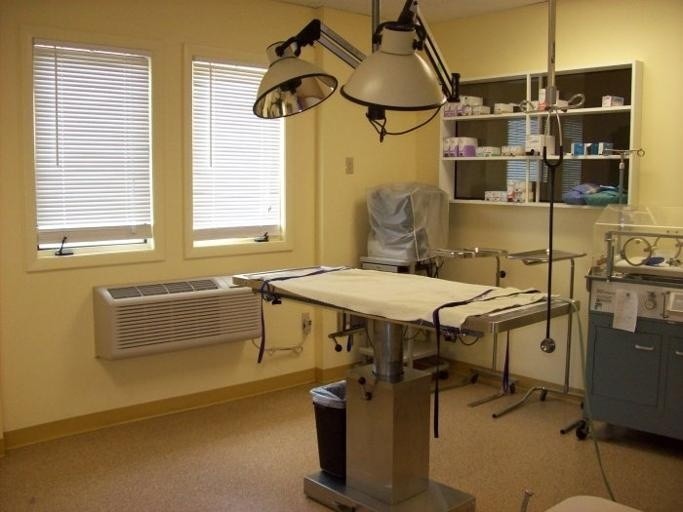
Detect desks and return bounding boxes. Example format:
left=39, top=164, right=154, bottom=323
left=230, top=266, right=580, bottom=512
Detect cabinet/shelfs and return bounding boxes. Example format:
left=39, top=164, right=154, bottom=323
left=439, top=58, right=637, bottom=211
left=577, top=273, right=681, bottom=444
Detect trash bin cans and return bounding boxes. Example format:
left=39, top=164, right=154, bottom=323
left=309, top=379, right=346, bottom=479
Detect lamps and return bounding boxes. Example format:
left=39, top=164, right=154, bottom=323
left=251, top=1, right=462, bottom=142
